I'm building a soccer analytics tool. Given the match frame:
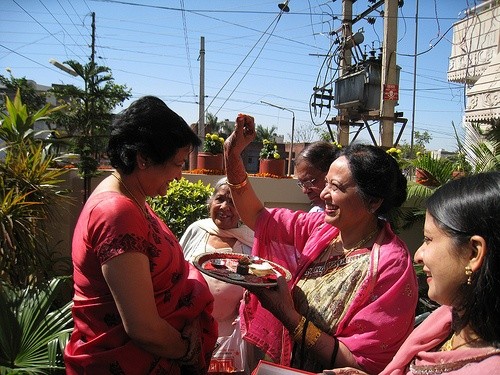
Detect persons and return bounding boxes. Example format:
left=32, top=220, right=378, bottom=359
left=63, top=96, right=219, bottom=375
left=178, top=178, right=254, bottom=375
left=293, top=140, right=339, bottom=213
left=224, top=113, right=418, bottom=375
left=318, top=171, right=500, bottom=375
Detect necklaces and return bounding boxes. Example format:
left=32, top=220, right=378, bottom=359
left=112, top=173, right=147, bottom=217
left=442, top=333, right=479, bottom=351
left=293, top=228, right=378, bottom=305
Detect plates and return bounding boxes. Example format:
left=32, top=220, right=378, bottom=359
left=227, top=273, right=245, bottom=281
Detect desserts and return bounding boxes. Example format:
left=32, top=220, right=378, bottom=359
left=236, top=256, right=249, bottom=275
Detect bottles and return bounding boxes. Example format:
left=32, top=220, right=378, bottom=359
left=237, top=260, right=251, bottom=274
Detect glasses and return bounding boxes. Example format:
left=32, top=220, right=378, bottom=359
left=296, top=171, right=325, bottom=189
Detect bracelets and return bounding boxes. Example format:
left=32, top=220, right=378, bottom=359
left=226, top=172, right=248, bottom=188
left=292, top=315, right=306, bottom=334
left=294, top=321, right=321, bottom=349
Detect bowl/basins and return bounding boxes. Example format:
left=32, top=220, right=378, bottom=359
left=212, top=259, right=225, bottom=267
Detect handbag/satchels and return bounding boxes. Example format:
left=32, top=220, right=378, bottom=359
left=205, top=316, right=250, bottom=375
left=251, top=319, right=339, bottom=375
